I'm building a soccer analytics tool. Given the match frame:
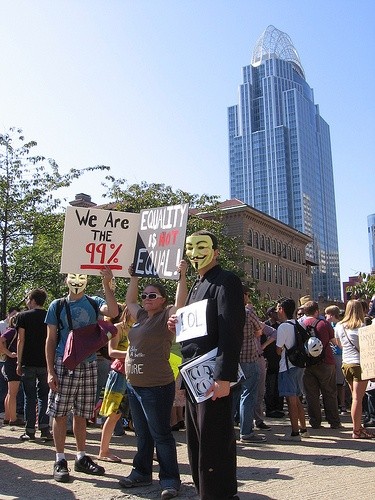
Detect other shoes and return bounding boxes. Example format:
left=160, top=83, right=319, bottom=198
left=364, top=420, right=375, bottom=428
left=20, top=432, right=35, bottom=439
left=177, top=420, right=186, bottom=429
left=170, top=423, right=180, bottom=431
left=40, top=433, right=53, bottom=442
left=242, top=411, right=345, bottom=441
left=340, top=406, right=348, bottom=416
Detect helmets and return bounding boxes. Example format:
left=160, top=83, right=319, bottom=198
left=305, top=337, right=323, bottom=357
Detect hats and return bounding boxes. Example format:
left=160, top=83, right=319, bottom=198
left=298, top=294, right=312, bottom=308
left=242, top=281, right=253, bottom=292
left=266, top=307, right=277, bottom=314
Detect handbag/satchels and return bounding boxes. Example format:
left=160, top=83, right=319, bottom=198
left=63, top=295, right=118, bottom=371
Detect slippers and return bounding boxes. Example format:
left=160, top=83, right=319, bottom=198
left=97, top=454, right=121, bottom=463
left=3, top=420, right=9, bottom=424
left=9, top=418, right=26, bottom=426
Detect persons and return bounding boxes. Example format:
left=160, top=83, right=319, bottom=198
left=276, top=297, right=311, bottom=441
left=14, top=289, right=53, bottom=442
left=119, top=259, right=187, bottom=499
left=325, top=295, right=375, bottom=439
left=0, top=307, right=25, bottom=419
left=0, top=312, right=27, bottom=426
left=97, top=304, right=158, bottom=462
left=236, top=283, right=285, bottom=441
left=168, top=229, right=246, bottom=500
left=294, top=295, right=344, bottom=428
left=66, top=302, right=126, bottom=436
left=44, top=265, right=119, bottom=483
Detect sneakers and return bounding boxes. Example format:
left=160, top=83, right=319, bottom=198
left=53, top=459, right=69, bottom=482
left=74, top=455, right=105, bottom=475
left=119, top=473, right=152, bottom=487
left=161, top=487, right=180, bottom=499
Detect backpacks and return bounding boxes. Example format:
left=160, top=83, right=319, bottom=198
left=283, top=319, right=310, bottom=368
left=0, top=329, right=17, bottom=362
left=298, top=317, right=324, bottom=362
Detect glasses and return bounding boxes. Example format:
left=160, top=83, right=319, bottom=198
left=142, top=293, right=163, bottom=300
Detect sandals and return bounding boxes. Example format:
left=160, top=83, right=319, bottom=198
left=352, top=430, right=373, bottom=439
left=360, top=426, right=375, bottom=438
